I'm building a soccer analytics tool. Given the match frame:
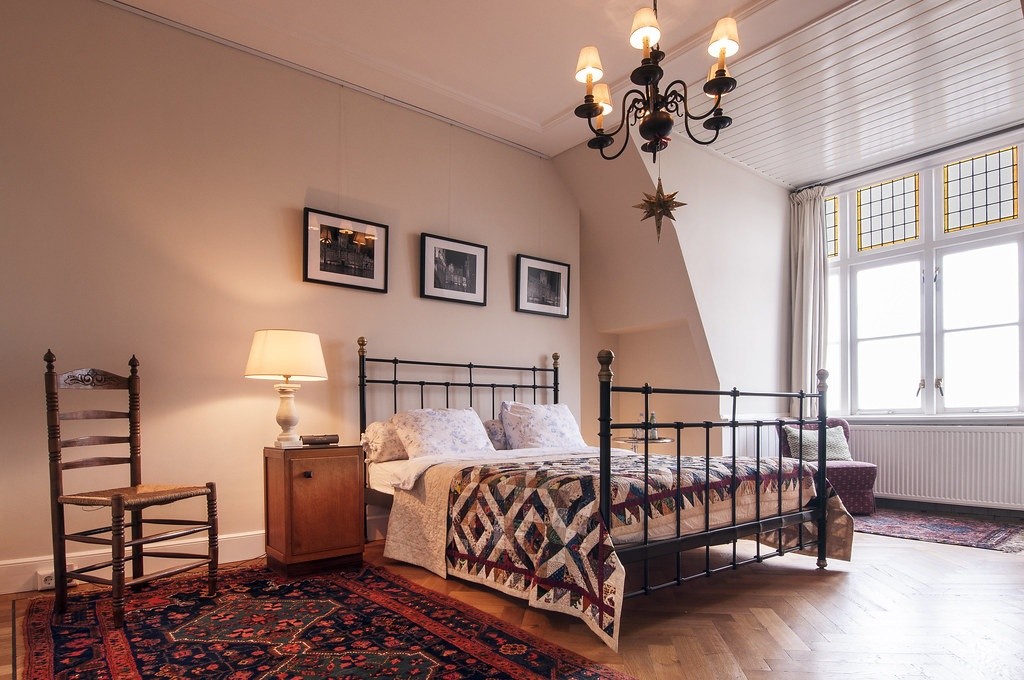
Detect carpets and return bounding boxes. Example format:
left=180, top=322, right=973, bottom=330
left=851, top=506, right=1024, bottom=553
left=21, top=562, right=639, bottom=680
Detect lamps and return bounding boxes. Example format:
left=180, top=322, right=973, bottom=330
left=574, top=0, right=741, bottom=241
left=244, top=328, right=329, bottom=448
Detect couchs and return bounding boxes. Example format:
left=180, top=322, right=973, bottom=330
left=775, top=417, right=878, bottom=515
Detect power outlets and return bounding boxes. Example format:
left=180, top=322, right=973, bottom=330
left=66, top=563, right=79, bottom=587
left=34, top=568, right=55, bottom=591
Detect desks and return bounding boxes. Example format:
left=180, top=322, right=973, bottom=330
left=614, top=435, right=674, bottom=453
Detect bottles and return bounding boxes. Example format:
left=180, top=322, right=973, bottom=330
left=637, top=412, right=646, bottom=439
left=650, top=413, right=657, bottom=439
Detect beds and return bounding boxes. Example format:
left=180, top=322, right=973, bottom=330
left=357, top=337, right=829, bottom=653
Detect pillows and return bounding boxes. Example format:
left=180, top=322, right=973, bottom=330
left=389, top=406, right=496, bottom=461
left=783, top=425, right=854, bottom=462
left=360, top=420, right=409, bottom=463
left=483, top=419, right=507, bottom=450
left=499, top=400, right=587, bottom=450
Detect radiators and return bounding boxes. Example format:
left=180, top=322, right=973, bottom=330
left=722, top=418, right=779, bottom=458
left=848, top=424, right=1024, bottom=511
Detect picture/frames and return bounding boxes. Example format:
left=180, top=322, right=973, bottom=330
left=303, top=207, right=389, bottom=294
left=515, top=254, right=570, bottom=318
left=419, top=231, right=488, bottom=307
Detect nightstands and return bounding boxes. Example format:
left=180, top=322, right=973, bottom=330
left=263, top=446, right=365, bottom=580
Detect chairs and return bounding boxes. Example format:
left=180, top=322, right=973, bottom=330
left=42, top=348, right=215, bottom=631
